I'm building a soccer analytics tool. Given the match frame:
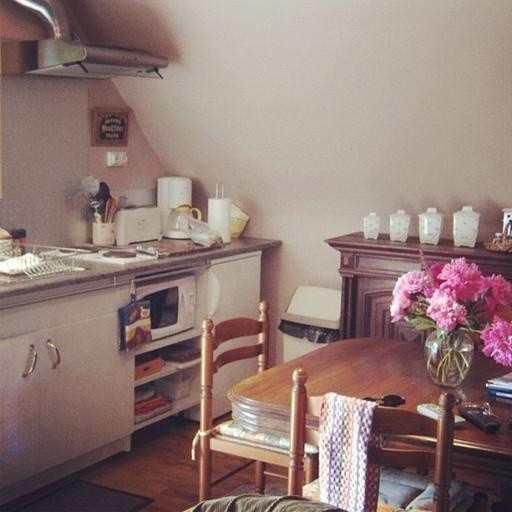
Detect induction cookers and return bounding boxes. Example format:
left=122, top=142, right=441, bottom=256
left=38, top=241, right=155, bottom=267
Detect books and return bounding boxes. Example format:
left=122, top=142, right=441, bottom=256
left=486, top=369, right=512, bottom=400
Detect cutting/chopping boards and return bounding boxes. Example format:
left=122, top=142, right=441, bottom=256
left=130, top=237, right=221, bottom=258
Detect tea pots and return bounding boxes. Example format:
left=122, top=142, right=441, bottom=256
left=164, top=204, right=202, bottom=240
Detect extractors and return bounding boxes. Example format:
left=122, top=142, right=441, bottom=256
left=0, top=0, right=170, bottom=79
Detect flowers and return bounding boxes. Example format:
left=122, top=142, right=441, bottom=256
left=390, top=256, right=511, bottom=384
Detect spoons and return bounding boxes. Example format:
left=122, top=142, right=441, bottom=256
left=90, top=201, right=101, bottom=224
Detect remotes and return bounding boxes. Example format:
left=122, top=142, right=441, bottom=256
left=458, top=399, right=501, bottom=433
left=415, top=400, right=466, bottom=430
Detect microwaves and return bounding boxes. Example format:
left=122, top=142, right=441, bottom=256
left=133, top=272, right=197, bottom=341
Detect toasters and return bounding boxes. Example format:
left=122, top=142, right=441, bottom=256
left=112, top=204, right=162, bottom=246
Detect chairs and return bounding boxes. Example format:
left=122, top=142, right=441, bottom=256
left=287, top=369, right=456, bottom=512
left=201, top=300, right=317, bottom=503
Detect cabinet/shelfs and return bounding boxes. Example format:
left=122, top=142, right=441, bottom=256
left=134, top=328, right=202, bottom=449
left=0, top=283, right=134, bottom=506
left=326, top=233, right=511, bottom=340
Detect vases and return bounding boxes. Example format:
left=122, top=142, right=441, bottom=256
left=423, top=329, right=474, bottom=388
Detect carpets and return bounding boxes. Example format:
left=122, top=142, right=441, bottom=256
left=12, top=479, right=156, bottom=511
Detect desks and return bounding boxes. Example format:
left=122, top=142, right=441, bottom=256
left=229, top=338, right=512, bottom=512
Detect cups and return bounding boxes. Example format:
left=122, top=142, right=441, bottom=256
left=452, top=206, right=482, bottom=249
left=12, top=228, right=26, bottom=255
left=388, top=209, right=413, bottom=244
left=363, top=211, right=382, bottom=241
left=417, top=207, right=447, bottom=246
